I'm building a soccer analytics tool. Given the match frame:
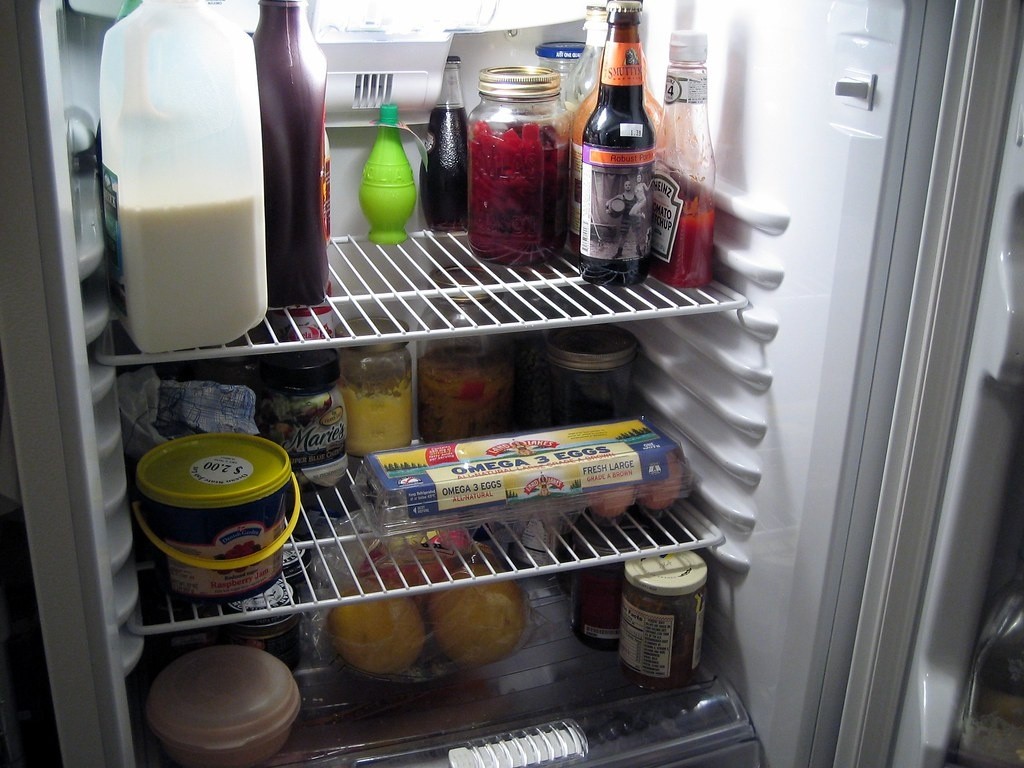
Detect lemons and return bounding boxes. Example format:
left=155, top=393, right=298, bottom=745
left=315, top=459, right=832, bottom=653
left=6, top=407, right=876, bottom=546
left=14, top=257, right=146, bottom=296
left=330, top=577, right=424, bottom=674
left=433, top=562, right=527, bottom=668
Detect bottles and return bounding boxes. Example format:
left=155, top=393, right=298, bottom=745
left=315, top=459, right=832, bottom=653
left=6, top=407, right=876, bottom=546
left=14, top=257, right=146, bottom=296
left=420, top=58, right=468, bottom=232
left=536, top=0, right=716, bottom=289
left=466, top=66, right=572, bottom=266
left=358, top=104, right=417, bottom=244
left=57, top=0, right=333, bottom=353
left=172, top=322, right=711, bottom=768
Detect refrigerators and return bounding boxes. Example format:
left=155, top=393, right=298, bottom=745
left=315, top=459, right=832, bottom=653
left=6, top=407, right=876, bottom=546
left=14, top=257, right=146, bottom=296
left=0, top=1, right=1023, bottom=768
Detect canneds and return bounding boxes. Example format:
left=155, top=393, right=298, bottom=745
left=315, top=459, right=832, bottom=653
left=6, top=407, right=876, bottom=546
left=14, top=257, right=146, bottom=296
left=250, top=348, right=349, bottom=494
left=567, top=520, right=708, bottom=691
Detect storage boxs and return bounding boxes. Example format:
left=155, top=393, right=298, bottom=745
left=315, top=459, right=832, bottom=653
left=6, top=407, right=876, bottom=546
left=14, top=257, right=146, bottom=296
left=348, top=420, right=698, bottom=553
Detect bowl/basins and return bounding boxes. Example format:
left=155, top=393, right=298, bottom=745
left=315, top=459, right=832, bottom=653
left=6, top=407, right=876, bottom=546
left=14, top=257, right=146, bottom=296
left=145, top=645, right=300, bottom=768
